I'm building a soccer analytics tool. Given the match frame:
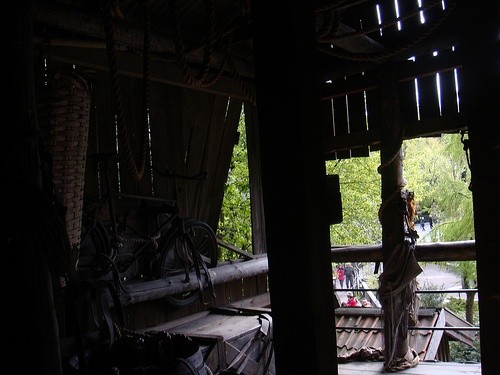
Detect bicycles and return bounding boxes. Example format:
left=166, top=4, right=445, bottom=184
left=81, top=151, right=218, bottom=308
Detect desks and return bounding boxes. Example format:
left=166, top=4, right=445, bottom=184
left=141, top=293, right=271, bottom=370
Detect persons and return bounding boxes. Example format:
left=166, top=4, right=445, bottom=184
left=339, top=291, right=372, bottom=308
left=336, top=264, right=358, bottom=288
